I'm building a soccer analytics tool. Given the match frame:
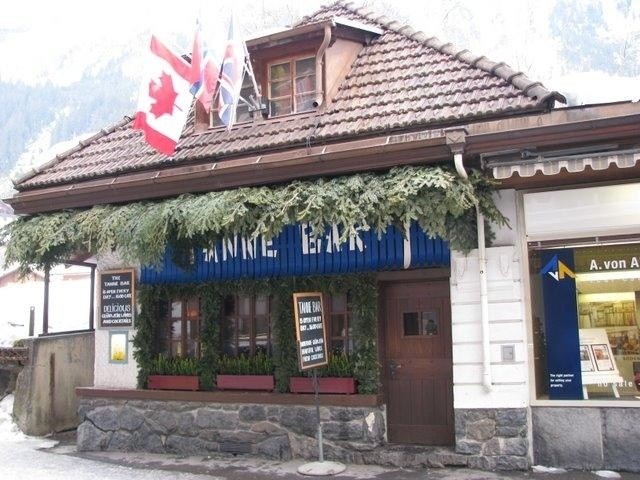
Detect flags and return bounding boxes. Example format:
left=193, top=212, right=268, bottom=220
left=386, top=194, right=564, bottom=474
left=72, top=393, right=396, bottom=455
left=133, top=34, right=193, bottom=158
left=277, top=76, right=314, bottom=109
left=216, top=19, right=247, bottom=133
left=188, top=19, right=225, bottom=114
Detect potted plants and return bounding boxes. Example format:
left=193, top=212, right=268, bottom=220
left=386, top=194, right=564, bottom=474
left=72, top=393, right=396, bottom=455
left=148, top=348, right=359, bottom=394
